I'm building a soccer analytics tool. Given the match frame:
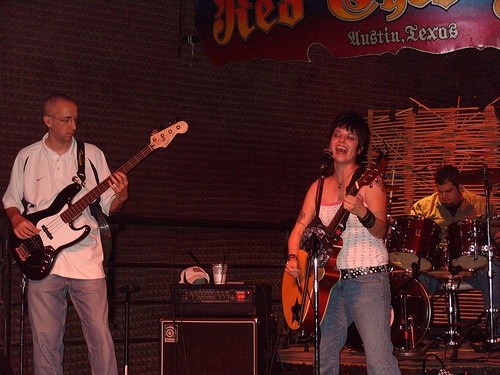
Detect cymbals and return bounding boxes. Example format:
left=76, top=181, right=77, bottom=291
left=448, top=165, right=500, bottom=185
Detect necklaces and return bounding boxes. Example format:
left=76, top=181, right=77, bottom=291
left=331, top=164, right=357, bottom=188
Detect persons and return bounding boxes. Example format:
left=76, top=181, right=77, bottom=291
left=282, top=112, right=402, bottom=375
left=0, top=95, right=129, bottom=375
left=408, top=164, right=500, bottom=348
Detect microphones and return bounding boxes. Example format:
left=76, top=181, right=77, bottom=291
left=320, top=148, right=333, bottom=170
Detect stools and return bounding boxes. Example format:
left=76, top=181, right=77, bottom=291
left=437, top=281, right=477, bottom=346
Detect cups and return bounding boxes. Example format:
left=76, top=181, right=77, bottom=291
left=212, top=263, right=227, bottom=285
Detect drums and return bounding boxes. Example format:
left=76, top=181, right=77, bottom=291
left=345, top=264, right=433, bottom=352
left=385, top=215, right=442, bottom=272
left=448, top=221, right=491, bottom=270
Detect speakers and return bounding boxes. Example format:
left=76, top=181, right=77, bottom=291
left=158, top=315, right=279, bottom=375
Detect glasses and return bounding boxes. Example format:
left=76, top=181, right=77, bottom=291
left=43, top=113, right=78, bottom=123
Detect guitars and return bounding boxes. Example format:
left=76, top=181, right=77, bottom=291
left=12, top=119, right=189, bottom=281
left=281, top=162, right=384, bottom=337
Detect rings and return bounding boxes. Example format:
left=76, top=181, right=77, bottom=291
left=116, top=185, right=120, bottom=187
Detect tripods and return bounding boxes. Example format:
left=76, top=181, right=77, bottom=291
left=419, top=171, right=500, bottom=362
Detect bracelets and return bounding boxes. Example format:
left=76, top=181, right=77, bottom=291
left=284, top=254, right=297, bottom=264
left=357, top=207, right=375, bottom=228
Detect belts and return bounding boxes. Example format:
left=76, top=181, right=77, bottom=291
left=341, top=265, right=394, bottom=280
